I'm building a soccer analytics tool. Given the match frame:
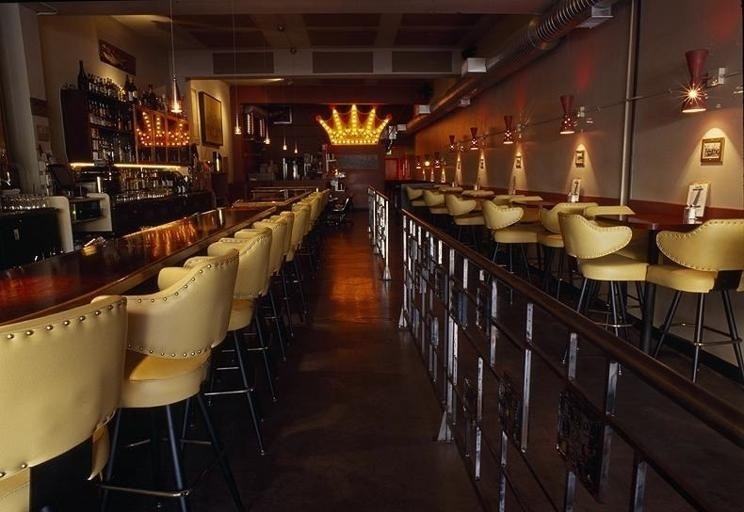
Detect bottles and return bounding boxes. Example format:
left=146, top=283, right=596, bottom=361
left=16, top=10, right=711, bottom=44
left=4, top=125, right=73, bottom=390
left=76, top=61, right=199, bottom=190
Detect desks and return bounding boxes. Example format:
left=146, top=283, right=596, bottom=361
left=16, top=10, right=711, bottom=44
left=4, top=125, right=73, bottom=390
left=595, top=215, right=707, bottom=325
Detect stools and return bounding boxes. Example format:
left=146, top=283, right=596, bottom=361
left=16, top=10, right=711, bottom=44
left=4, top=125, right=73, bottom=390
left=480, top=200, right=552, bottom=304
left=644, top=217, right=744, bottom=386
left=536, top=199, right=600, bottom=301
left=89, top=247, right=244, bottom=510
left=400, top=185, right=547, bottom=256
left=557, top=211, right=649, bottom=376
left=2, top=294, right=129, bottom=511
left=217, top=188, right=352, bottom=366
left=181, top=227, right=278, bottom=458
left=583, top=203, right=646, bottom=321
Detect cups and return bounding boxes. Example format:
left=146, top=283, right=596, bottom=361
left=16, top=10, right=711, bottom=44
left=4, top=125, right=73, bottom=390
left=0, top=193, right=49, bottom=211
left=115, top=191, right=173, bottom=204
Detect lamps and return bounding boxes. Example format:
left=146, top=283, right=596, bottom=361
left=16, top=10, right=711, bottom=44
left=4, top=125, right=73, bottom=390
left=397, top=49, right=709, bottom=175
left=170, top=0, right=183, bottom=113
left=234, top=0, right=298, bottom=154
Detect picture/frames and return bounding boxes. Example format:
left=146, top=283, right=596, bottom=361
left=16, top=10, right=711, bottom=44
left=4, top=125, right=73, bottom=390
left=700, top=138, right=725, bottom=162
left=97, top=40, right=138, bottom=77
left=200, top=91, right=223, bottom=147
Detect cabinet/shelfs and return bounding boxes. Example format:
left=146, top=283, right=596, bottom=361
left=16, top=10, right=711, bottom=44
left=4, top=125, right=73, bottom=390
left=0, top=88, right=212, bottom=270
